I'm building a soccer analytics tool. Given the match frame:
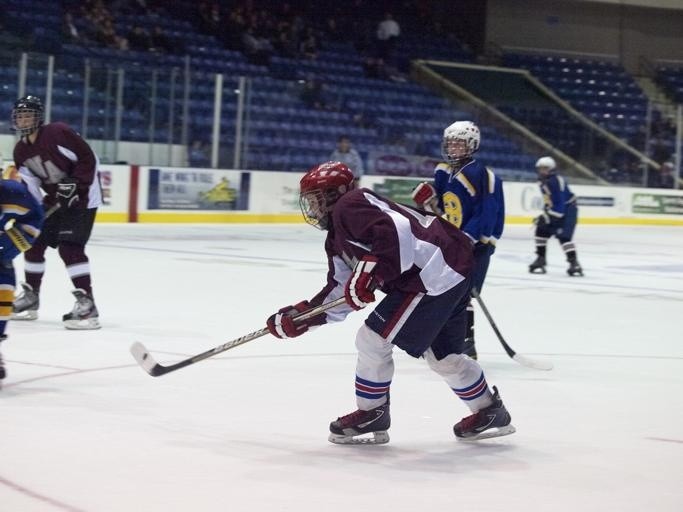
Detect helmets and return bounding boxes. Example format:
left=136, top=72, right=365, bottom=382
left=442, top=121, right=480, bottom=167
left=11, top=95, right=44, bottom=136
left=298, top=161, right=354, bottom=230
left=537, top=156, right=557, bottom=176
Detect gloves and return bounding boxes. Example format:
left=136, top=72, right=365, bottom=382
left=412, top=181, right=437, bottom=204
left=267, top=300, right=326, bottom=339
left=344, top=255, right=380, bottom=311
left=55, top=177, right=80, bottom=213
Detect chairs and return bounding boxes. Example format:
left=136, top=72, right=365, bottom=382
left=0, top=1, right=682, bottom=189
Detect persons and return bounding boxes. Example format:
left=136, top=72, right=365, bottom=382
left=526, top=157, right=585, bottom=277
left=0, top=179, right=47, bottom=378
left=328, top=133, right=363, bottom=176
left=185, top=139, right=207, bottom=167
left=416, top=119, right=504, bottom=360
left=298, top=77, right=326, bottom=105
left=322, top=0, right=452, bottom=81
left=611, top=117, right=682, bottom=189
left=57, top=1, right=320, bottom=57
left=267, top=161, right=512, bottom=440
left=9, top=95, right=103, bottom=329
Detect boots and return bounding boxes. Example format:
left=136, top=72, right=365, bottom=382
left=63, top=288, right=99, bottom=320
left=14, top=282, right=39, bottom=312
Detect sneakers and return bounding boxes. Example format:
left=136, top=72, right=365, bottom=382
left=453, top=402, right=510, bottom=437
left=530, top=257, right=546, bottom=268
left=330, top=404, right=390, bottom=435
left=567, top=261, right=581, bottom=272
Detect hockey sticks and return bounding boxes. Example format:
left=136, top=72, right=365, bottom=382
left=471, top=285, right=552, bottom=370
left=130, top=275, right=378, bottom=376
